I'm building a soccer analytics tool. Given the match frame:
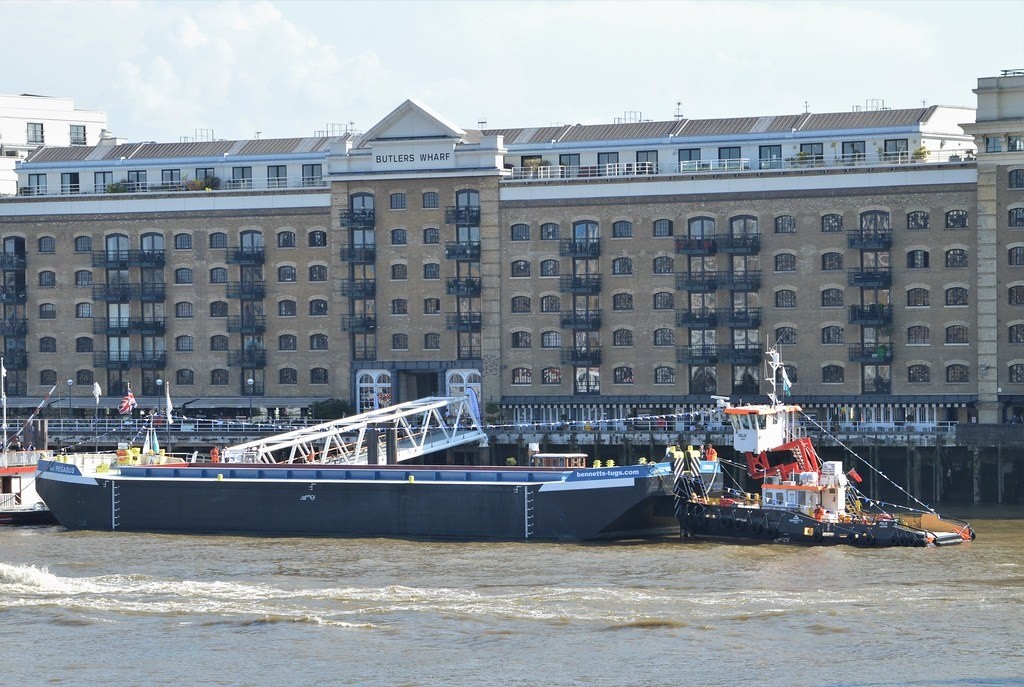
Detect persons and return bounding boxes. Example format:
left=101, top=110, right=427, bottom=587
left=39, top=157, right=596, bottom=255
left=676, top=444, right=681, bottom=451
left=27, top=442, right=33, bottom=451
left=445, top=409, right=450, bottom=418
left=16, top=442, right=25, bottom=451
left=813, top=504, right=824, bottom=522
left=699, top=445, right=706, bottom=460
left=210, top=445, right=229, bottom=464
left=666, top=443, right=672, bottom=457
left=826, top=510, right=831, bottom=523
left=0, top=441, right=3, bottom=452
left=706, top=444, right=717, bottom=460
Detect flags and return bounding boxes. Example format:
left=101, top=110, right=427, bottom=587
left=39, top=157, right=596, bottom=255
left=166, top=385, right=173, bottom=424
left=118, top=388, right=137, bottom=414
left=142, top=431, right=150, bottom=454
left=848, top=469, right=862, bottom=482
left=783, top=367, right=791, bottom=397
left=153, top=429, right=159, bottom=455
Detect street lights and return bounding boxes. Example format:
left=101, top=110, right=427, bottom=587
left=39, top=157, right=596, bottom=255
left=156, top=379, right=163, bottom=418
left=247, top=379, right=254, bottom=431
left=67, top=379, right=73, bottom=427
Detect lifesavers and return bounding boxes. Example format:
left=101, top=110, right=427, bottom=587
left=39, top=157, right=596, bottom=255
left=863, top=535, right=874, bottom=545
left=696, top=515, right=706, bottom=526
left=33, top=502, right=45, bottom=511
left=766, top=527, right=778, bottom=538
left=718, top=517, right=730, bottom=529
left=812, top=531, right=822, bottom=542
left=734, top=520, right=744, bottom=530
left=889, top=537, right=917, bottom=547
left=749, top=523, right=761, bottom=535
left=846, top=533, right=855, bottom=544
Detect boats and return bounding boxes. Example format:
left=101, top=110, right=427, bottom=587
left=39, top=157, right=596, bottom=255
left=34, top=429, right=723, bottom=542
left=0, top=357, right=220, bottom=523
left=670, top=325, right=975, bottom=548
